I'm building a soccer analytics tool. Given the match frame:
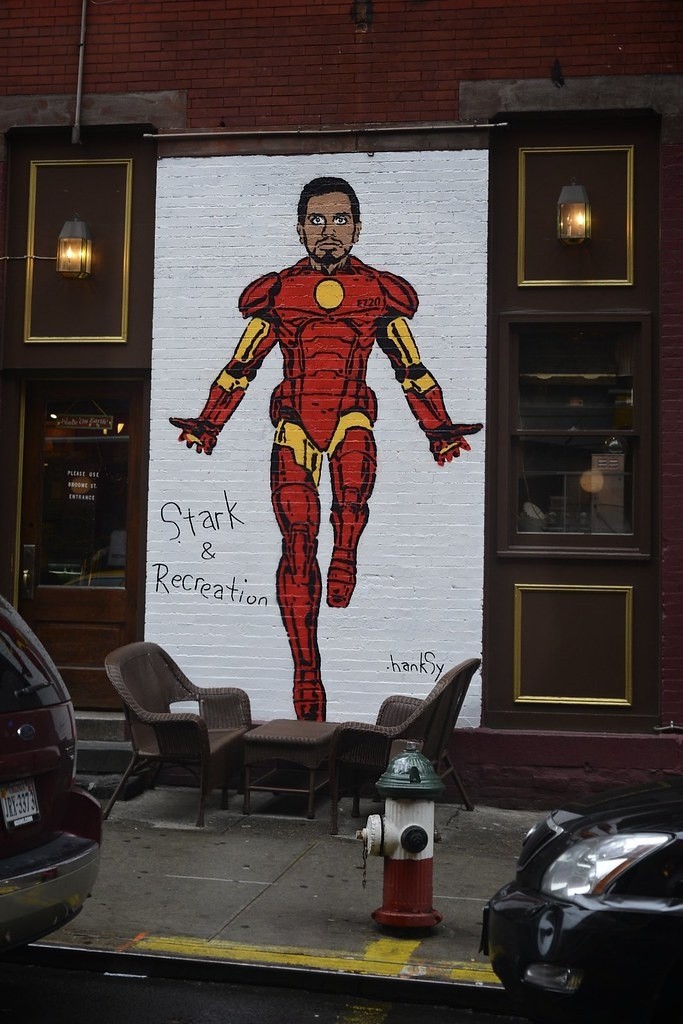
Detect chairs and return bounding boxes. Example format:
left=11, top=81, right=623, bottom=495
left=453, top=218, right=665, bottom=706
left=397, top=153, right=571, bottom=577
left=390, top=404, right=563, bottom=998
left=327, top=655, right=484, bottom=834
left=100, top=637, right=255, bottom=828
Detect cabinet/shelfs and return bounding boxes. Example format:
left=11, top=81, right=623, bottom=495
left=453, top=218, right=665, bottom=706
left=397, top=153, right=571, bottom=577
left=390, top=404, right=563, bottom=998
left=2, top=368, right=147, bottom=710
left=492, top=313, right=661, bottom=563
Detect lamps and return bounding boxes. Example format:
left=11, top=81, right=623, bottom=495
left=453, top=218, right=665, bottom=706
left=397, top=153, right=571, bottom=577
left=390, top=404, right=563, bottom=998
left=54, top=211, right=96, bottom=279
left=558, top=174, right=594, bottom=249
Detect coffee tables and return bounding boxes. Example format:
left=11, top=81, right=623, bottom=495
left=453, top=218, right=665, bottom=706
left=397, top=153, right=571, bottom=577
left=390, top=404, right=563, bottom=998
left=240, top=718, right=342, bottom=821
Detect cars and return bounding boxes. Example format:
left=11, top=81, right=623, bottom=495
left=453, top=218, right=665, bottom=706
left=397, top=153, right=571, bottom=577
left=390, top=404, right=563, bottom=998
left=478, top=797, right=682, bottom=1024
left=0, top=586, right=105, bottom=960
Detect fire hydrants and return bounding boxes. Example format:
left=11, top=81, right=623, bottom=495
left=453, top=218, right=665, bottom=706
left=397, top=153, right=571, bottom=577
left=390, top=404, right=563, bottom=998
left=355, top=741, right=445, bottom=929
